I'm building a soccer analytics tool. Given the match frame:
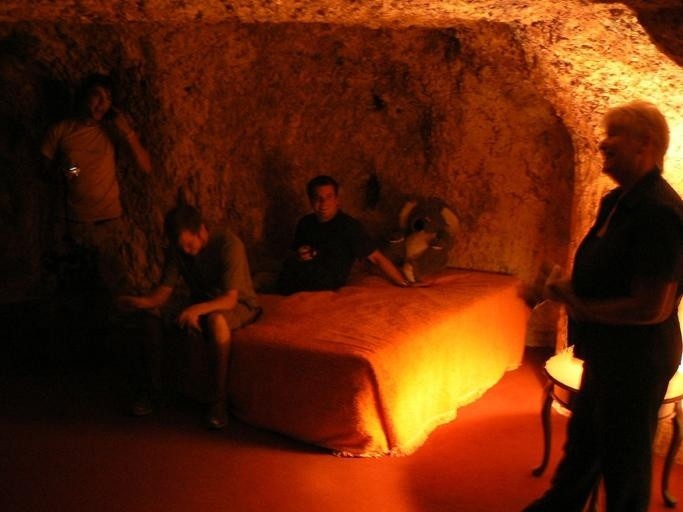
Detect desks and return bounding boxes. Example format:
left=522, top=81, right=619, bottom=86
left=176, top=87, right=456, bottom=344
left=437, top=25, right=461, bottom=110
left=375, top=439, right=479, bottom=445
left=530, top=346, right=682, bottom=512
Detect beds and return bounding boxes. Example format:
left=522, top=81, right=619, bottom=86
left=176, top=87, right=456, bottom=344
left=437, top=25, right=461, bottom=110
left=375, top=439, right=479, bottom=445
left=123, top=257, right=537, bottom=463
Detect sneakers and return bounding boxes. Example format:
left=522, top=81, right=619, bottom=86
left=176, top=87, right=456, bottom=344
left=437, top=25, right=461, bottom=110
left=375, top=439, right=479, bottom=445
left=208, top=405, right=229, bottom=431
left=131, top=390, right=164, bottom=417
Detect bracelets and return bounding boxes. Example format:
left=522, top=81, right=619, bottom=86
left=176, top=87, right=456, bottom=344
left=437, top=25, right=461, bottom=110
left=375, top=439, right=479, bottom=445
left=122, top=130, right=134, bottom=145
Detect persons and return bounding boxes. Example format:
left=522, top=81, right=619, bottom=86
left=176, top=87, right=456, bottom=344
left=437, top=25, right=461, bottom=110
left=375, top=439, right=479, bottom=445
left=39, top=75, right=155, bottom=400
left=523, top=102, right=682, bottom=511
left=252, top=175, right=415, bottom=296
left=115, top=203, right=263, bottom=431
